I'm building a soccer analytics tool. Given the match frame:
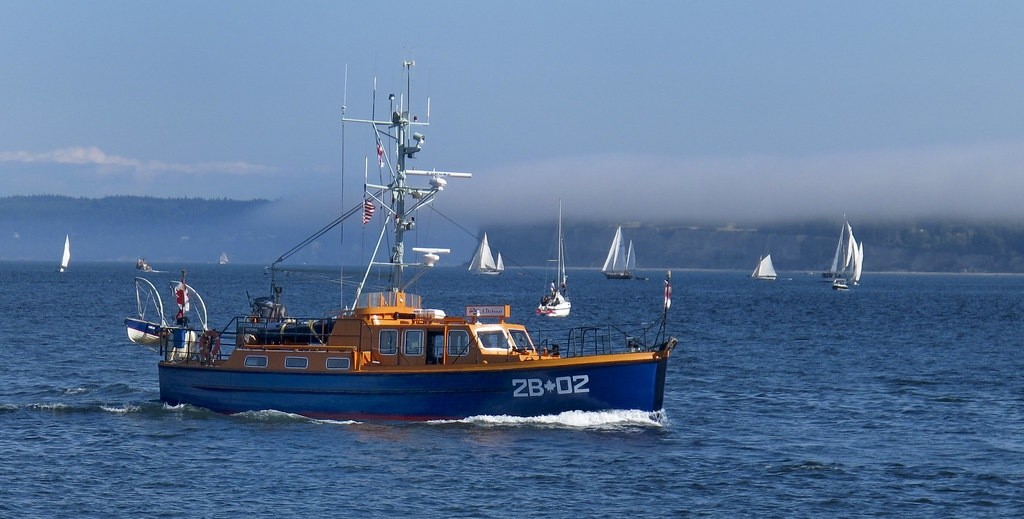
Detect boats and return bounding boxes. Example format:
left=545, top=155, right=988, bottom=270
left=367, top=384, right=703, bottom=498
left=158, top=60, right=678, bottom=423
left=136, top=257, right=152, bottom=272
left=124, top=317, right=171, bottom=345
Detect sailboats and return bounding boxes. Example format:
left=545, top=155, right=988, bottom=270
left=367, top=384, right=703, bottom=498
left=56, top=234, right=70, bottom=273
left=467, top=232, right=504, bottom=275
left=535, top=200, right=571, bottom=318
left=832, top=236, right=863, bottom=291
left=600, top=226, right=635, bottom=279
left=820, top=214, right=852, bottom=281
left=752, top=254, right=777, bottom=280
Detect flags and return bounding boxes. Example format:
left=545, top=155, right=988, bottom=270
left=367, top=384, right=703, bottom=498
left=363, top=199, right=375, bottom=224
left=175, top=282, right=190, bottom=318
left=665, top=281, right=672, bottom=309
left=376, top=143, right=385, bottom=168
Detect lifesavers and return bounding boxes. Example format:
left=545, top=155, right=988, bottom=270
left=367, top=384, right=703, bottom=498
left=198, top=330, right=221, bottom=359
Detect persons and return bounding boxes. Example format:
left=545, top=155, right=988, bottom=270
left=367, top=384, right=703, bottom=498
left=540, top=280, right=564, bottom=306
